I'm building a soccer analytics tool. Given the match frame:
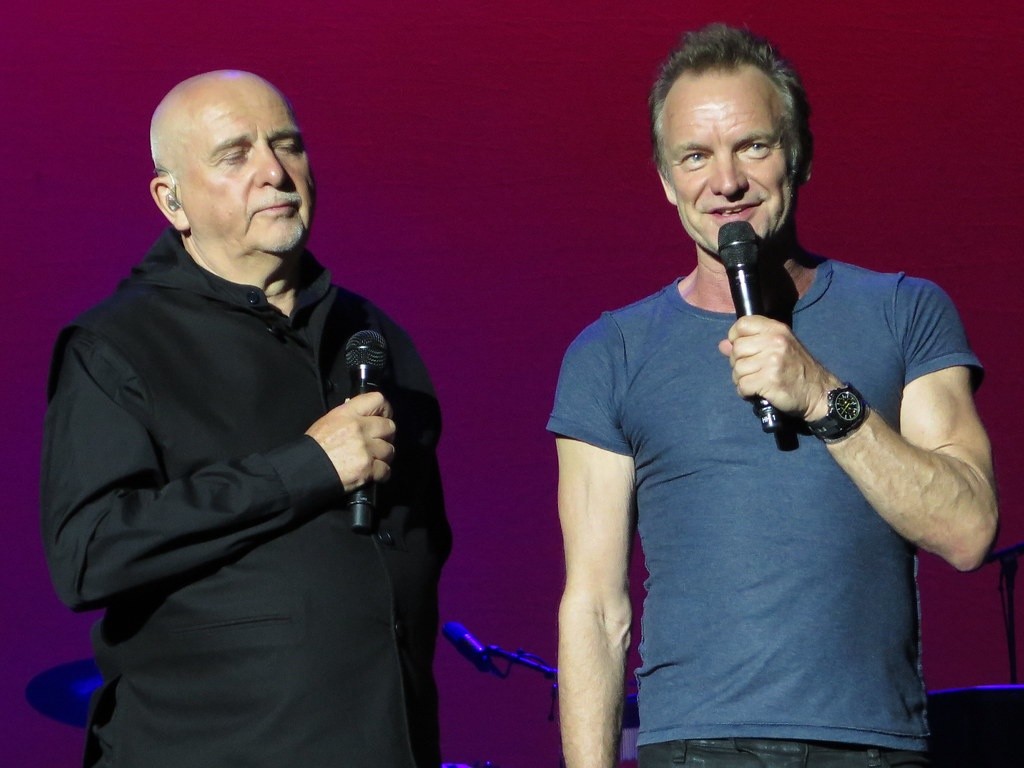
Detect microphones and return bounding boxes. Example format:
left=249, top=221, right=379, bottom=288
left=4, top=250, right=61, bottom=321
left=345, top=330, right=388, bottom=533
left=443, top=622, right=505, bottom=681
left=718, top=221, right=783, bottom=433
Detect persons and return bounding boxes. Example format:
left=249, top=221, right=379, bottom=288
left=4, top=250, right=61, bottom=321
left=542, top=21, right=1001, bottom=768
left=42, top=70, right=456, bottom=768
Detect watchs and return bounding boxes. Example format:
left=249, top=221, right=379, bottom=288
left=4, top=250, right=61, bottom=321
left=805, top=380, right=868, bottom=440
